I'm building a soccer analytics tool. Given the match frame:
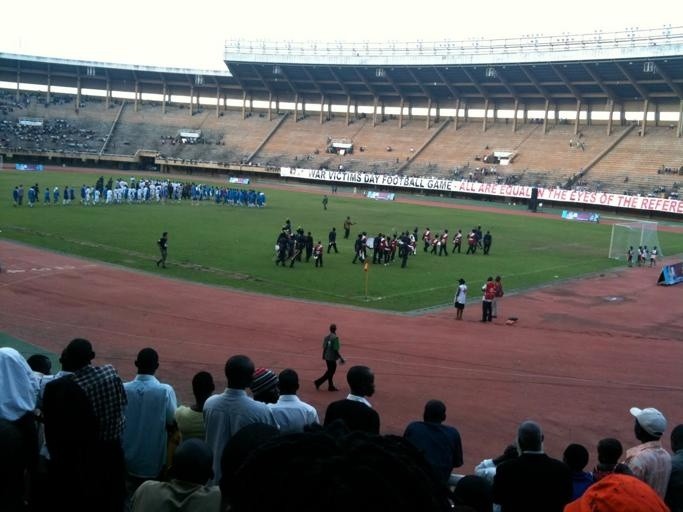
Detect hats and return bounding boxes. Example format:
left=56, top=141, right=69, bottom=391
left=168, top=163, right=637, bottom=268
left=629, top=406, right=672, bottom=438
left=249, top=368, right=280, bottom=396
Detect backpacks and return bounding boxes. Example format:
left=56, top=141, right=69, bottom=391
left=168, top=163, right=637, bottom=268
left=495, top=282, right=504, bottom=297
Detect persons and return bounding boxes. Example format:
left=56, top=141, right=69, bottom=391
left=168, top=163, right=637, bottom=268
left=454, top=275, right=467, bottom=321
left=490, top=276, right=502, bottom=319
left=479, top=277, right=496, bottom=322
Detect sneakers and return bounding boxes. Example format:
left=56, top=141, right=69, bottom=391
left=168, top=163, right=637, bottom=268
left=271, top=256, right=326, bottom=269
left=351, top=248, right=491, bottom=267
left=12, top=200, right=267, bottom=208
left=454, top=315, right=498, bottom=323
left=156, top=261, right=168, bottom=269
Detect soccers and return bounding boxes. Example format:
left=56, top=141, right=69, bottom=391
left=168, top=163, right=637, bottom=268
left=554, top=275, right=558, bottom=279
